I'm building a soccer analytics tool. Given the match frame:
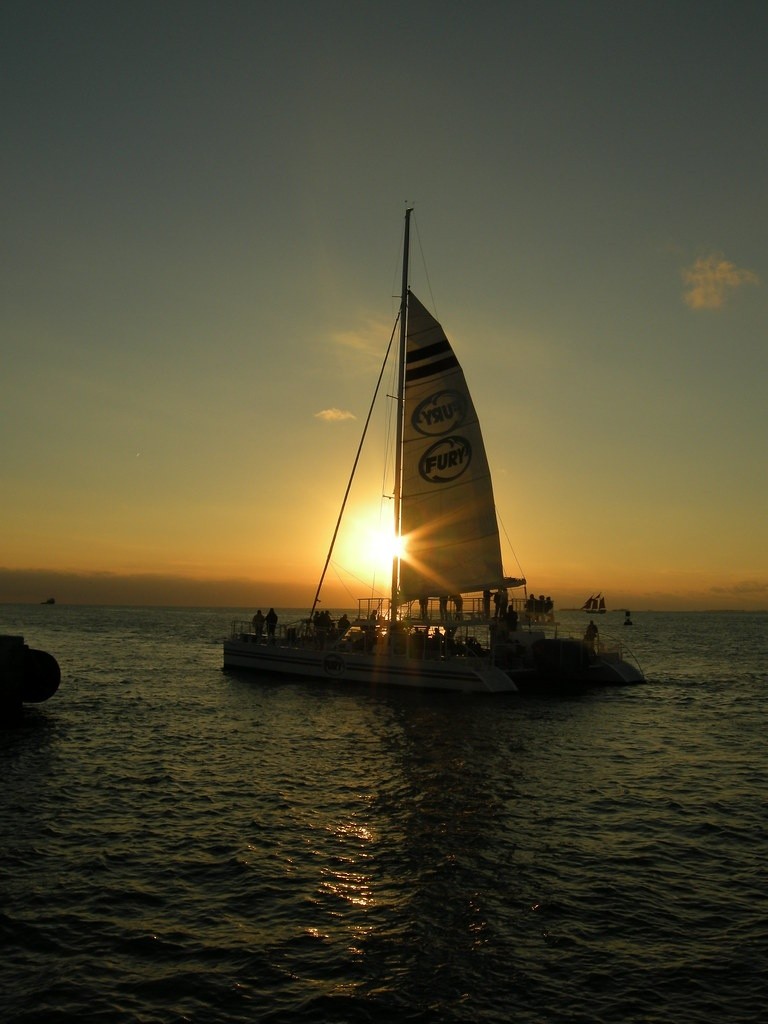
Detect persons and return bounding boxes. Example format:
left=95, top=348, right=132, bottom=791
left=312, top=585, right=554, bottom=659
left=252, top=610, right=265, bottom=643
left=265, top=608, right=278, bottom=645
left=584, top=620, right=598, bottom=654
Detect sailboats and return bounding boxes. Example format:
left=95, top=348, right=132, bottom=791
left=217, top=206, right=655, bottom=699
